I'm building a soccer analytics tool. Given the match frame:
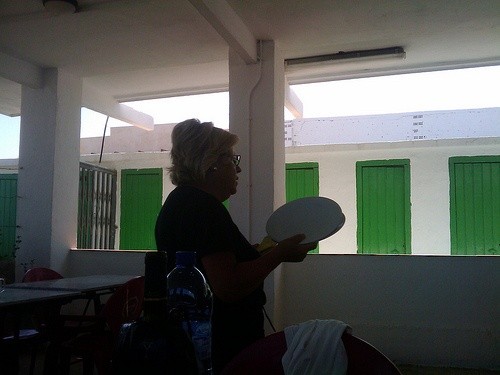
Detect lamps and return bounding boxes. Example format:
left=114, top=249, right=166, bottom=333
left=284, top=46, right=407, bottom=73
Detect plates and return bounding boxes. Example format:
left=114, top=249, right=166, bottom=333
left=266, top=196, right=345, bottom=246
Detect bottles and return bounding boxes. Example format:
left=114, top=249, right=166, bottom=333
left=167, top=250, right=214, bottom=375
left=118, top=252, right=198, bottom=375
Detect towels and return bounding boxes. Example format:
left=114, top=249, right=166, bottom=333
left=282, top=319, right=353, bottom=375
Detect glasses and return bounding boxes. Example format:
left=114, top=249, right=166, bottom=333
left=231, top=154, right=241, bottom=166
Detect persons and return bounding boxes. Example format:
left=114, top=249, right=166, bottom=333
left=155, top=120, right=316, bottom=363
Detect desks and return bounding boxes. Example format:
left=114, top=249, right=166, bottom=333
left=0, top=288, right=79, bottom=375
left=0, top=274, right=140, bottom=375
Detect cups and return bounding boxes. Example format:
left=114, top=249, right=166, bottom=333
left=0, top=278, right=6, bottom=293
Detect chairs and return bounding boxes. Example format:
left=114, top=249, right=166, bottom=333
left=218, top=331, right=402, bottom=375
left=22, top=267, right=144, bottom=375
